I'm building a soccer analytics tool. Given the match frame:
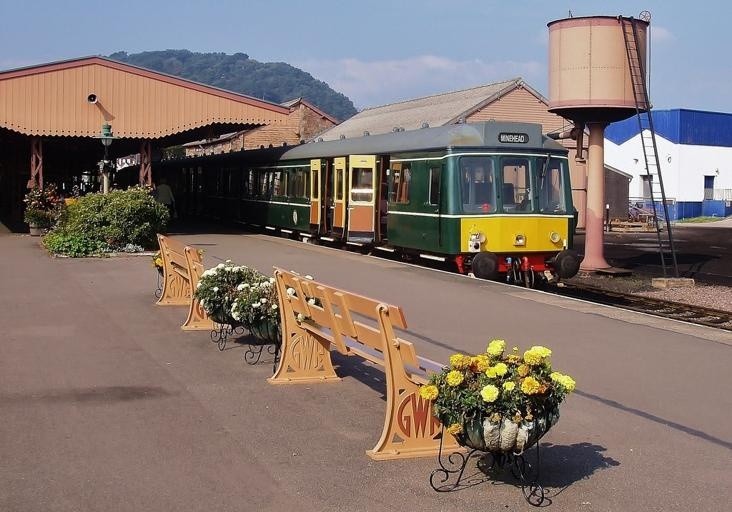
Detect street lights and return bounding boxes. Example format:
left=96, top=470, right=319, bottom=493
left=92, top=122, right=120, bottom=196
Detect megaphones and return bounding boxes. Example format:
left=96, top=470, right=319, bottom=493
left=87, top=93, right=98, bottom=104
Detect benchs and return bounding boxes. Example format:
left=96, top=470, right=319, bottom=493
left=155, top=232, right=224, bottom=332
left=264, top=263, right=466, bottom=462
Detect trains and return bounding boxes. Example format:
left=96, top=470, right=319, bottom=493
left=116, top=115, right=585, bottom=289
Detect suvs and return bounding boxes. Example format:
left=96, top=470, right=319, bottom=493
left=629, top=200, right=663, bottom=222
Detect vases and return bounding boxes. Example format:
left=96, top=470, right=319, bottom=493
left=28, top=220, right=46, bottom=236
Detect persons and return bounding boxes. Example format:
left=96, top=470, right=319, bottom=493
left=157, top=178, right=175, bottom=223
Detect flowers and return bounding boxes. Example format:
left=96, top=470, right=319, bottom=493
left=226, top=270, right=317, bottom=327
left=151, top=246, right=206, bottom=277
left=194, top=256, right=258, bottom=317
left=417, top=337, right=577, bottom=439
left=22, top=182, right=62, bottom=226
